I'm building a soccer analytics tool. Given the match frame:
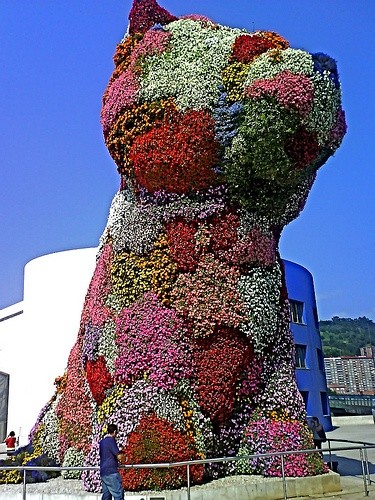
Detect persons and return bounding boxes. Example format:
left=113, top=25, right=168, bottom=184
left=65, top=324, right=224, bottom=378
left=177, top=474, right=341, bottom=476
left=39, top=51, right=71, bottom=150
left=311, top=417, right=326, bottom=459
left=4, top=431, right=16, bottom=456
left=99, top=424, right=127, bottom=500
